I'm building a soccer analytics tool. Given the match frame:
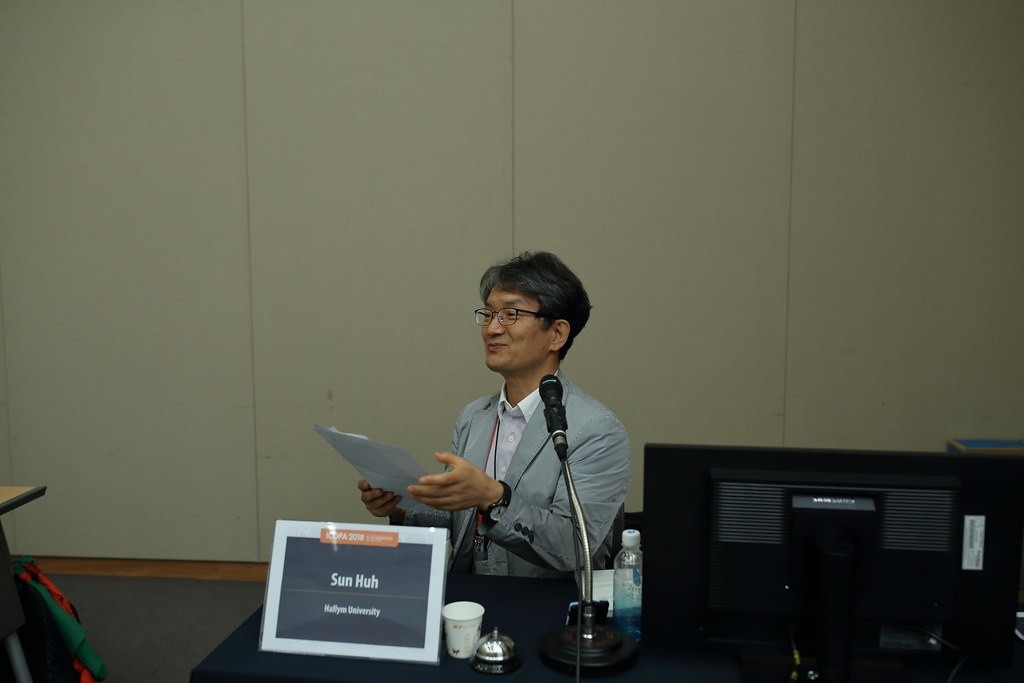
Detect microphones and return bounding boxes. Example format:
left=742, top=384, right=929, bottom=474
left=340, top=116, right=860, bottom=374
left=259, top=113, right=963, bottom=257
left=538, top=373, right=570, bottom=462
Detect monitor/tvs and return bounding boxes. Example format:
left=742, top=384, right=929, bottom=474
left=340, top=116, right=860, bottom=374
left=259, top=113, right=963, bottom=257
left=639, top=442, right=1024, bottom=683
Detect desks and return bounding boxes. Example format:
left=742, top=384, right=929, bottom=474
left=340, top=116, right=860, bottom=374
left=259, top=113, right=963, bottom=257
left=193, top=571, right=748, bottom=683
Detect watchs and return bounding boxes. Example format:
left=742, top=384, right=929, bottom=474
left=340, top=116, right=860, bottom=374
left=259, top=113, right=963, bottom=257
left=484, top=480, right=513, bottom=528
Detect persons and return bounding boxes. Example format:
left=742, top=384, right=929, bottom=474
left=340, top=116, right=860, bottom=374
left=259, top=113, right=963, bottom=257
left=356, top=253, right=630, bottom=579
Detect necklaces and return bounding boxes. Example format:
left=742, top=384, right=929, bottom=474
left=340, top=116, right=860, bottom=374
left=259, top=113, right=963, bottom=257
left=493, top=406, right=504, bottom=480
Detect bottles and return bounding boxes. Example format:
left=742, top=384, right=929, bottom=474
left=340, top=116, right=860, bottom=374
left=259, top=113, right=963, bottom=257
left=612, top=529, right=643, bottom=643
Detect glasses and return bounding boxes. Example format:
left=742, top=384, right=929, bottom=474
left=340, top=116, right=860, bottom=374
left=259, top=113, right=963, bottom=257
left=474, top=307, right=540, bottom=326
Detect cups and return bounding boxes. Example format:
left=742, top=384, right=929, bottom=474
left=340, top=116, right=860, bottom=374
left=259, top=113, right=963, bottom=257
left=442, top=602, right=485, bottom=659
